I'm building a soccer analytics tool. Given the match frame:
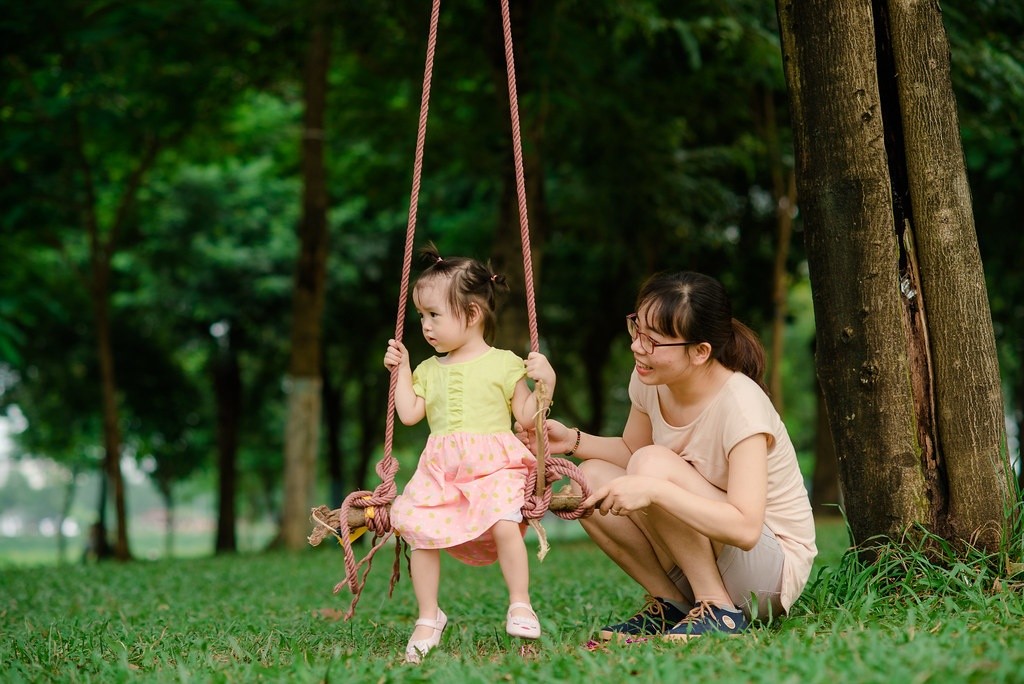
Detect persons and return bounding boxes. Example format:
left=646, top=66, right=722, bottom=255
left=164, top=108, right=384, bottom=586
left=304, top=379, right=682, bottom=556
left=384, top=240, right=556, bottom=662
left=516, top=266, right=818, bottom=638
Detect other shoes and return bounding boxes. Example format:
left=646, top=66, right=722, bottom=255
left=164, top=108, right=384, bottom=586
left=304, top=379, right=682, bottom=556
left=406, top=607, right=447, bottom=663
left=506, top=602, right=541, bottom=639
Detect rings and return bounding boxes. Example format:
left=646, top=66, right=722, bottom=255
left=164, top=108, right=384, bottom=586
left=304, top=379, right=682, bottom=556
left=606, top=506, right=619, bottom=512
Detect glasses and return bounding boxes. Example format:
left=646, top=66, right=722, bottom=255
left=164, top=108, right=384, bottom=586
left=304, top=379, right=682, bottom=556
left=626, top=313, right=699, bottom=354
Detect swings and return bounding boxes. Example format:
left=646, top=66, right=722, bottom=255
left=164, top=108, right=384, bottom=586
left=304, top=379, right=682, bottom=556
left=307, top=0, right=612, bottom=547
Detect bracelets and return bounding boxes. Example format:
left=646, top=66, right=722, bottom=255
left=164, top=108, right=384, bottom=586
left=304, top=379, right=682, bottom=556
left=564, top=427, right=580, bottom=456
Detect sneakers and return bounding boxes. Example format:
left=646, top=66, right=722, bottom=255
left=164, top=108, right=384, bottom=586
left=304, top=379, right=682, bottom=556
left=599, top=595, right=688, bottom=642
left=664, top=600, right=751, bottom=642
